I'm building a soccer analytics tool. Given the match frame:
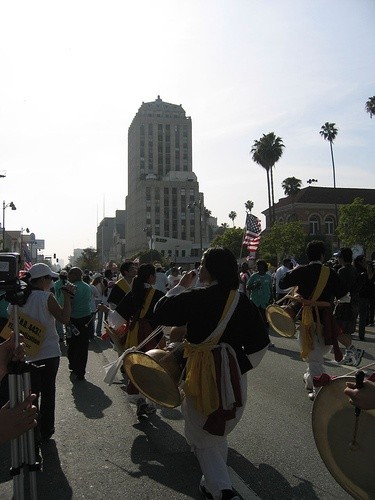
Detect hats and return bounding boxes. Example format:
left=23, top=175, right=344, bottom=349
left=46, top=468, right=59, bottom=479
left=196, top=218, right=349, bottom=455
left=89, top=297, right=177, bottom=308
left=170, top=267, right=179, bottom=272
left=60, top=270, right=68, bottom=276
left=93, top=272, right=103, bottom=280
left=27, top=262, right=59, bottom=281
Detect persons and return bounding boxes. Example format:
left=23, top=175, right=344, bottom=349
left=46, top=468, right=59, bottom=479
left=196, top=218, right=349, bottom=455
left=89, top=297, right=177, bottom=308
left=0, top=240, right=375, bottom=500
left=154, top=246, right=271, bottom=500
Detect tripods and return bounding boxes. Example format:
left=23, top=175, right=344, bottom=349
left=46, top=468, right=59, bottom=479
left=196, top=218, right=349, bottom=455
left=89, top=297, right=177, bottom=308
left=3, top=284, right=39, bottom=500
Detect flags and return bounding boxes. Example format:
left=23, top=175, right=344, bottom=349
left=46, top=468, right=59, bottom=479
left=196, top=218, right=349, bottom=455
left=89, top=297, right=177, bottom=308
left=242, top=214, right=262, bottom=252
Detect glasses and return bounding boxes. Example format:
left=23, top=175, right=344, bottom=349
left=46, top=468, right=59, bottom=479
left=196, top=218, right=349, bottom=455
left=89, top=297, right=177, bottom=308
left=44, top=275, right=52, bottom=280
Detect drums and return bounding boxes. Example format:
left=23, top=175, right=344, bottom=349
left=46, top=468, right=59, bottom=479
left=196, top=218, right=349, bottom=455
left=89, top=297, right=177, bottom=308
left=124, top=324, right=187, bottom=410
left=265, top=285, right=304, bottom=337
left=102, top=321, right=128, bottom=353
left=312, top=368, right=375, bottom=500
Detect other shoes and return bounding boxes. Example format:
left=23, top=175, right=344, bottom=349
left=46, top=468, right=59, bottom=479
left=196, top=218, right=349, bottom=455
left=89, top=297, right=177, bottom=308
left=303, top=372, right=322, bottom=401
left=198, top=481, right=242, bottom=500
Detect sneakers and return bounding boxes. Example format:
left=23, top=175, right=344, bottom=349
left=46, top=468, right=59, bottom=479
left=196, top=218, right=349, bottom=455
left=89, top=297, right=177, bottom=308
left=342, top=345, right=356, bottom=363
left=352, top=349, right=365, bottom=366
left=136, top=398, right=156, bottom=416
